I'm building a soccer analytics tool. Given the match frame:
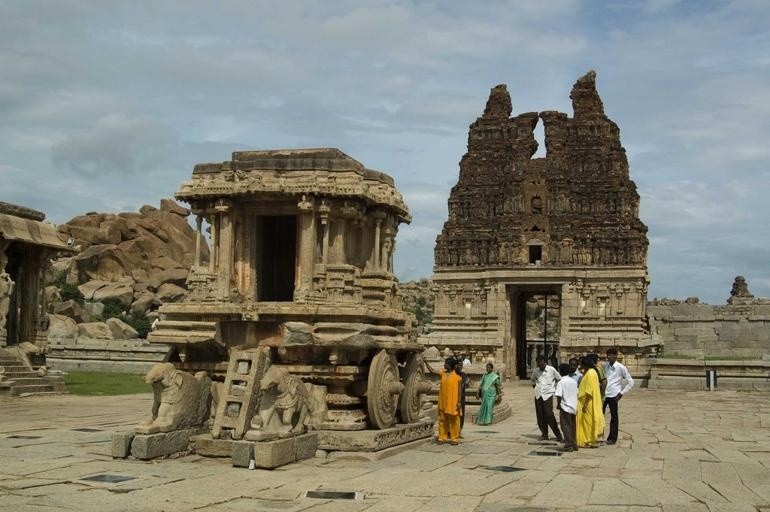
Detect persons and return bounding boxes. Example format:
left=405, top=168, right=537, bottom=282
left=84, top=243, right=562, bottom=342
left=475, top=363, right=501, bottom=425
left=531, top=355, right=563, bottom=442
left=453, top=352, right=471, bottom=368
left=555, top=363, right=579, bottom=452
left=602, top=348, right=634, bottom=445
left=422, top=357, right=461, bottom=446
left=569, top=354, right=607, bottom=448
left=448, top=359, right=471, bottom=439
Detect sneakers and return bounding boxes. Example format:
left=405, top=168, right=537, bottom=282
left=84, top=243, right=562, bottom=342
left=537, top=435, right=615, bottom=452
